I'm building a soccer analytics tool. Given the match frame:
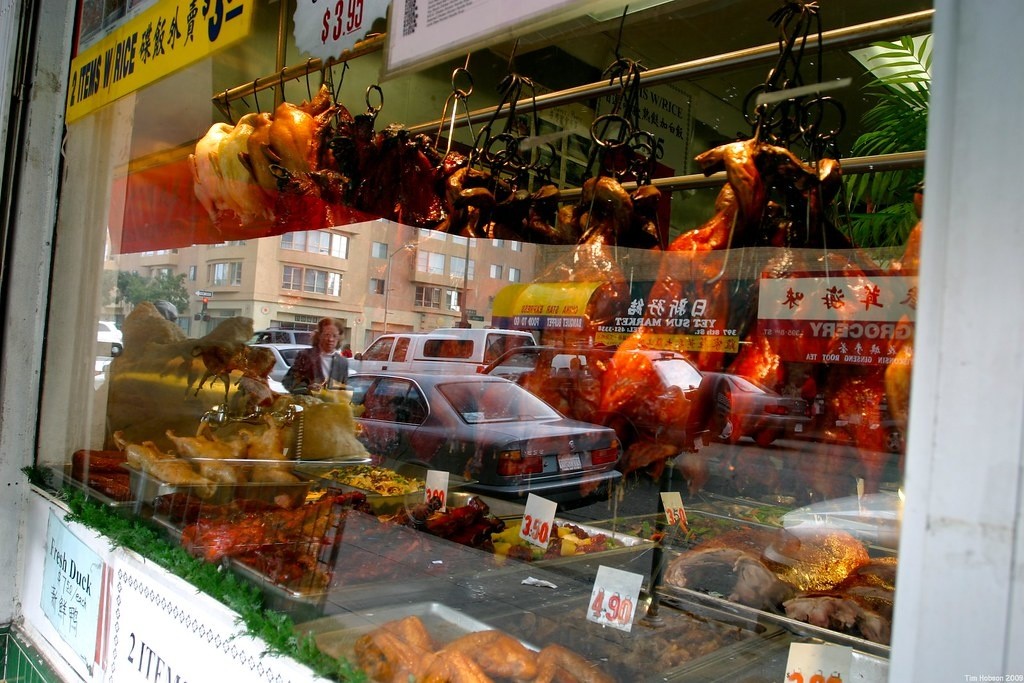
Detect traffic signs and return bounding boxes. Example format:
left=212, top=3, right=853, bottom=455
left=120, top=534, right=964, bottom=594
left=196, top=290, right=214, bottom=298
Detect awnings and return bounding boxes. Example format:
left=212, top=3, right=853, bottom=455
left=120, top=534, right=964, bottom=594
left=491, top=283, right=616, bottom=331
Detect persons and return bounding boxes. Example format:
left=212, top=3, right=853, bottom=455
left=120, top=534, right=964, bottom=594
left=283, top=317, right=349, bottom=399
left=797, top=371, right=816, bottom=420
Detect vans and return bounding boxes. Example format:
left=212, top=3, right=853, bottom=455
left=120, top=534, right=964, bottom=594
left=341, top=327, right=540, bottom=392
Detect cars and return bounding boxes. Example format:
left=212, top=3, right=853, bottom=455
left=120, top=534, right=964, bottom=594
left=263, top=374, right=293, bottom=396
left=93, top=321, right=125, bottom=389
left=692, top=369, right=813, bottom=447
left=245, top=342, right=359, bottom=383
left=343, top=372, right=624, bottom=509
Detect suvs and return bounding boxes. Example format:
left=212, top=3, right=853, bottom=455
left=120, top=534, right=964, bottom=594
left=247, top=327, right=318, bottom=346
left=478, top=344, right=712, bottom=467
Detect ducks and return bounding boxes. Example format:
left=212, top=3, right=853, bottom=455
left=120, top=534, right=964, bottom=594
left=114, top=91, right=926, bottom=683
